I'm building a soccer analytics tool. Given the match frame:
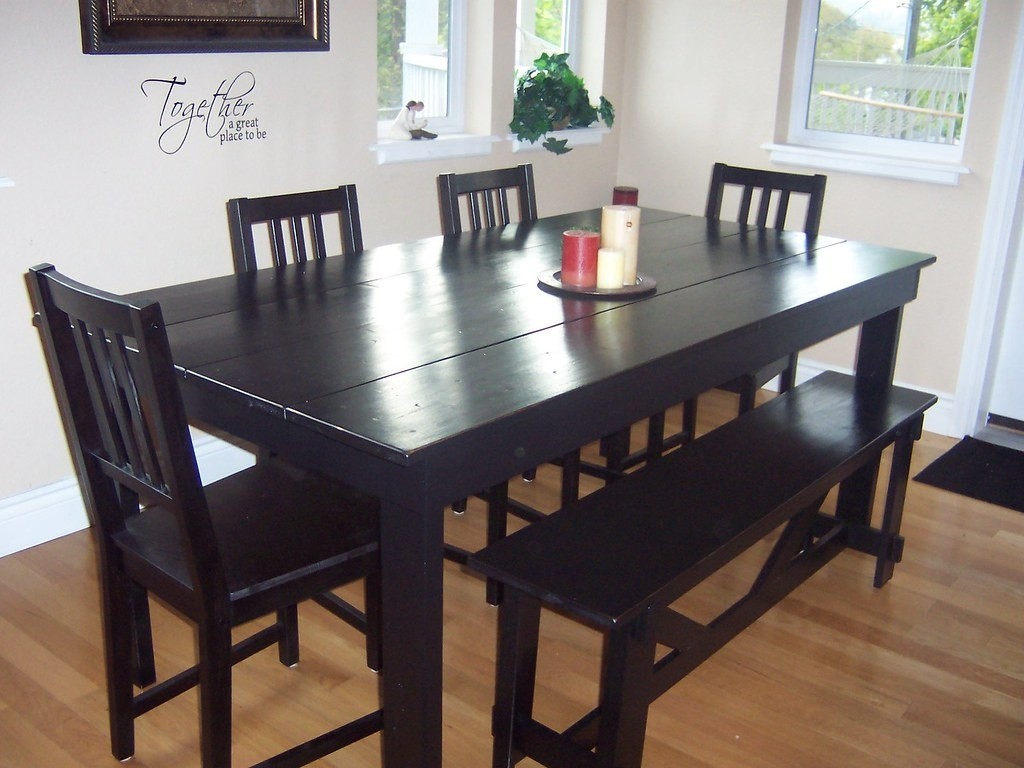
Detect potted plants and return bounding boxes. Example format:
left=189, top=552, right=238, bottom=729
left=508, top=52, right=615, bottom=155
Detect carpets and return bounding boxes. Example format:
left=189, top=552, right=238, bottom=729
left=912, top=434, right=1024, bottom=514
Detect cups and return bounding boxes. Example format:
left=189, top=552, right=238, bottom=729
left=601, top=205, right=641, bottom=286
left=597, top=248, right=622, bottom=289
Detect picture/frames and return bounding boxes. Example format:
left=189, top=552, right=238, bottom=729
left=78, top=0, right=330, bottom=54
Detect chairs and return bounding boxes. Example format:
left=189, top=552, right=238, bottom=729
left=227, top=164, right=666, bottom=672
left=606, top=163, right=827, bottom=471
left=29, top=264, right=383, bottom=768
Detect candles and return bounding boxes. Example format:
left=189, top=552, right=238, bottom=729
left=560, top=186, right=642, bottom=289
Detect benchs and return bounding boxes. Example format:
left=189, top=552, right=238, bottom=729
left=467, top=370, right=938, bottom=768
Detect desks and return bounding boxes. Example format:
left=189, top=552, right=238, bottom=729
left=33, top=204, right=937, bottom=768
